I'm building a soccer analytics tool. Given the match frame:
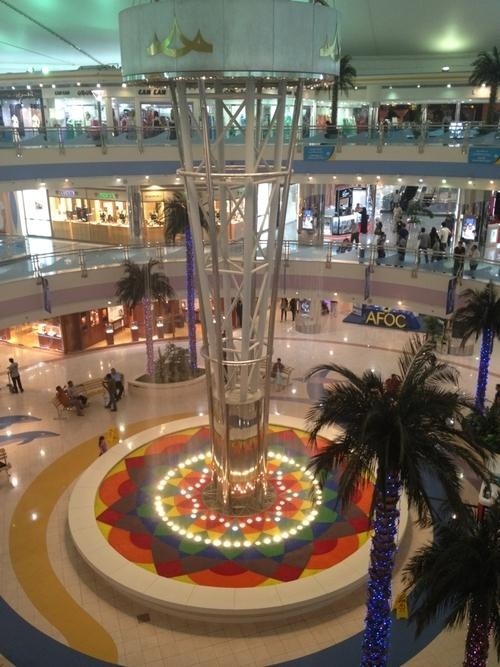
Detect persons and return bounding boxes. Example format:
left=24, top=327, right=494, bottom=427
left=385, top=374, right=403, bottom=402
left=236, top=299, right=243, bottom=326
left=103, top=367, right=124, bottom=412
left=278, top=297, right=297, bottom=322
left=440, top=111, right=453, bottom=133
left=338, top=186, right=481, bottom=278
left=89, top=108, right=177, bottom=142
left=7, top=358, right=24, bottom=395
left=321, top=300, right=329, bottom=313
left=270, top=358, right=284, bottom=379
left=98, top=435, right=109, bottom=456
left=489, top=382, right=500, bottom=411
left=56, top=381, right=87, bottom=417
left=324, top=119, right=337, bottom=136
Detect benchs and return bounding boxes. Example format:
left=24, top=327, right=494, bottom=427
left=0, top=447, right=12, bottom=477
left=50, top=374, right=126, bottom=418
left=261, top=361, right=295, bottom=389
